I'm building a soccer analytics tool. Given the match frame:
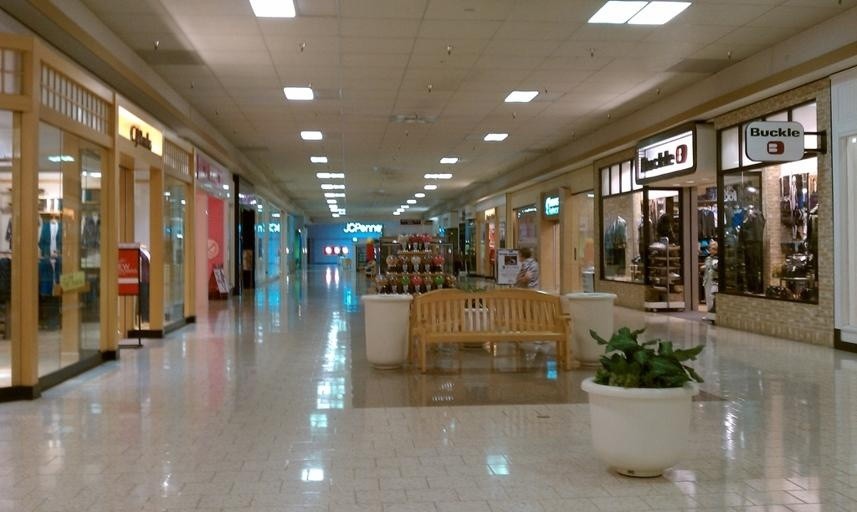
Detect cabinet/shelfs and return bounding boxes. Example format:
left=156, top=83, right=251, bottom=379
left=645, top=237, right=685, bottom=312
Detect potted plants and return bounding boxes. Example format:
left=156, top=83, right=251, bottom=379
left=580, top=327, right=705, bottom=478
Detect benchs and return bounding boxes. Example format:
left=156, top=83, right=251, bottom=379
left=411, top=288, right=572, bottom=374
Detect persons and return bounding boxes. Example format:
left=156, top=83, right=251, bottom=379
left=700, top=241, right=719, bottom=321
left=517, top=248, right=540, bottom=288
left=743, top=210, right=765, bottom=294
left=611, top=215, right=627, bottom=269
